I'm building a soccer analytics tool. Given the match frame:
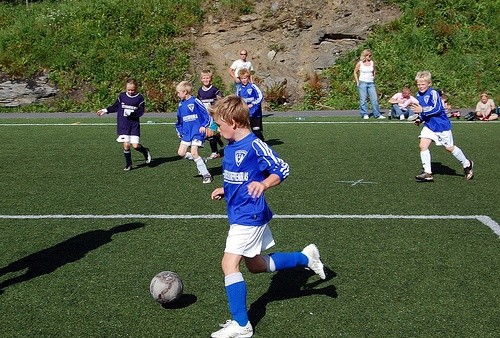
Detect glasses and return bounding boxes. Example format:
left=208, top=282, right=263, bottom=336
left=240, top=54, right=246, bottom=55
left=369, top=55, right=372, bottom=57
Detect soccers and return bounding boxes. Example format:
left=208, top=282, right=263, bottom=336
left=149, top=271, right=183, bottom=303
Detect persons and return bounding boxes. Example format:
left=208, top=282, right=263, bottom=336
left=475, top=94, right=498, bottom=120
left=210, top=95, right=327, bottom=338
left=353, top=49, right=387, bottom=119
left=237, top=69, right=265, bottom=142
left=388, top=86, right=420, bottom=121
left=196, top=69, right=225, bottom=160
left=409, top=71, right=474, bottom=182
left=97, top=78, right=152, bottom=171
left=174, top=81, right=212, bottom=185
left=229, top=49, right=254, bottom=97
left=436, top=90, right=453, bottom=117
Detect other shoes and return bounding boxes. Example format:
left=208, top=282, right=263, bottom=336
left=363, top=115, right=369, bottom=119
left=377, top=115, right=386, bottom=120
left=400, top=115, right=405, bottom=121
left=408, top=113, right=419, bottom=121
left=464, top=111, right=476, bottom=121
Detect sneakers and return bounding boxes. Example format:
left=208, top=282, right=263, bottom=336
left=124, top=164, right=132, bottom=171
left=415, top=171, right=433, bottom=182
left=301, top=244, right=326, bottom=280
left=464, top=160, right=474, bottom=180
left=210, top=319, right=253, bottom=338
left=207, top=152, right=220, bottom=159
left=202, top=173, right=212, bottom=184
left=144, top=148, right=152, bottom=164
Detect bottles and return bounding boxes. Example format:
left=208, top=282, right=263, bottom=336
left=388, top=110, right=392, bottom=120
left=146, top=121, right=157, bottom=124
left=296, top=117, right=305, bottom=120
